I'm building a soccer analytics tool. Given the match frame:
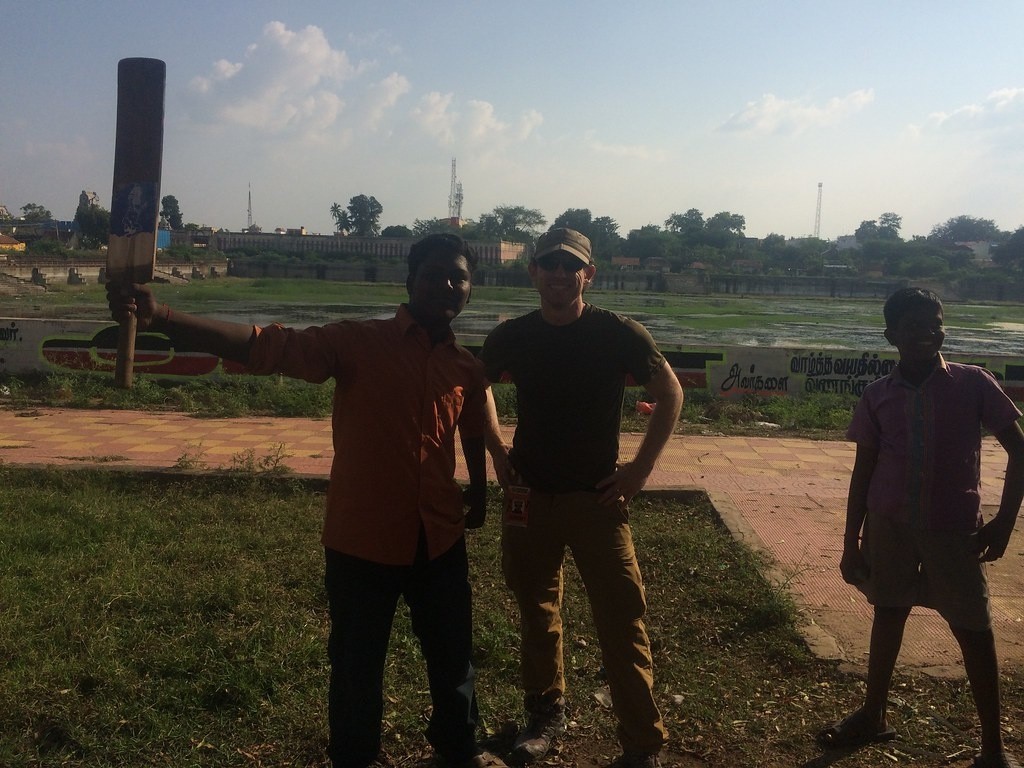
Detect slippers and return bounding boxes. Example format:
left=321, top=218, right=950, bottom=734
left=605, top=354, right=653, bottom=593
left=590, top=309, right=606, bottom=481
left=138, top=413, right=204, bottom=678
left=975, top=751, right=1012, bottom=768
left=818, top=708, right=897, bottom=748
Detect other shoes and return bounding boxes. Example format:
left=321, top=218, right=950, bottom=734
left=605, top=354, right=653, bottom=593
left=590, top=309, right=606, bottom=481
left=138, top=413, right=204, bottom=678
left=433, top=747, right=510, bottom=768
left=623, top=749, right=662, bottom=768
left=511, top=697, right=567, bottom=763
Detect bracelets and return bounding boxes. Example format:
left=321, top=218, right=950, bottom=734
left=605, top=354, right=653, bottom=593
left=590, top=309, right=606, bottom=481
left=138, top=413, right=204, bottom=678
left=161, top=303, right=174, bottom=331
left=844, top=533, right=860, bottom=540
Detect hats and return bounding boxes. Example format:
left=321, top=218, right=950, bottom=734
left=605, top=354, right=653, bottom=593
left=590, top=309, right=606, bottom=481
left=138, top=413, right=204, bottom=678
left=532, top=228, right=594, bottom=267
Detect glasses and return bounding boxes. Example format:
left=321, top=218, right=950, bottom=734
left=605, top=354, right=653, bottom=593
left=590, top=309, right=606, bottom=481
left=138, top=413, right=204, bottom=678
left=536, top=255, right=590, bottom=272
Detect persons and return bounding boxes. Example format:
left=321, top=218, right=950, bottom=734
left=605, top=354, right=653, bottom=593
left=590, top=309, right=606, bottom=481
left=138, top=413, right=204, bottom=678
left=107, top=232, right=488, bottom=768
left=478, top=227, right=684, bottom=768
left=821, top=287, right=1024, bottom=767
